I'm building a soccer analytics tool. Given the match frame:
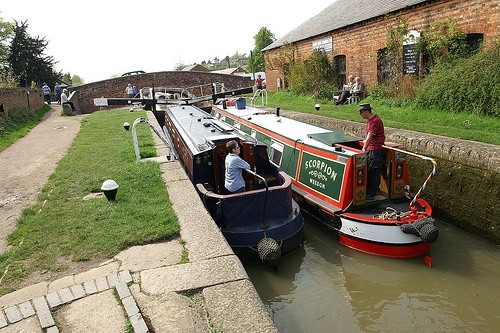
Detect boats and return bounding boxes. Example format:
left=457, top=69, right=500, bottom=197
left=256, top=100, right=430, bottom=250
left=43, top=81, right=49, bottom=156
left=209, top=96, right=440, bottom=259
left=163, top=103, right=306, bottom=269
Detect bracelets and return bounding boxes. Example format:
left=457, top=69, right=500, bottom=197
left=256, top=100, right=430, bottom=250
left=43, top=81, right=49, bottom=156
left=363, top=146, right=367, bottom=149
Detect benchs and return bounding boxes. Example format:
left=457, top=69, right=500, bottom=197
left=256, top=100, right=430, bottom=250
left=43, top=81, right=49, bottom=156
left=330, top=84, right=362, bottom=106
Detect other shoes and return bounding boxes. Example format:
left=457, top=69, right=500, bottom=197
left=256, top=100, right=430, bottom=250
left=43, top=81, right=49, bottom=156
left=335, top=100, right=343, bottom=103
left=72, top=108, right=78, bottom=111
left=366, top=194, right=375, bottom=200
left=336, top=103, right=339, bottom=105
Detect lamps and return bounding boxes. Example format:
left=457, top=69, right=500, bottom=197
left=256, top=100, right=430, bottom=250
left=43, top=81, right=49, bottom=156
left=122, top=122, right=130, bottom=131
left=314, top=104, right=321, bottom=111
left=100, top=179, right=119, bottom=202
left=129, top=107, right=134, bottom=112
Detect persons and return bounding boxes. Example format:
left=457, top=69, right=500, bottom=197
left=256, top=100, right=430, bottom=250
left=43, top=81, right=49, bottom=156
left=224, top=140, right=251, bottom=193
left=54, top=83, right=63, bottom=104
left=125, top=83, right=133, bottom=98
left=335, top=75, right=363, bottom=106
left=42, top=82, right=52, bottom=104
left=255, top=75, right=265, bottom=96
left=357, top=104, right=385, bottom=200
left=133, top=85, right=140, bottom=98
left=61, top=89, right=78, bottom=112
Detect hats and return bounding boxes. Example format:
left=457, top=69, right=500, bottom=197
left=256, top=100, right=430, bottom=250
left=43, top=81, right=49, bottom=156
left=63, top=88, right=67, bottom=93
left=357, top=103, right=372, bottom=111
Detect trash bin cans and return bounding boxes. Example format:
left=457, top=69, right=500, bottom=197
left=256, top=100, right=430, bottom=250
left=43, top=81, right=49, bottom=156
left=235, top=100, right=246, bottom=109
